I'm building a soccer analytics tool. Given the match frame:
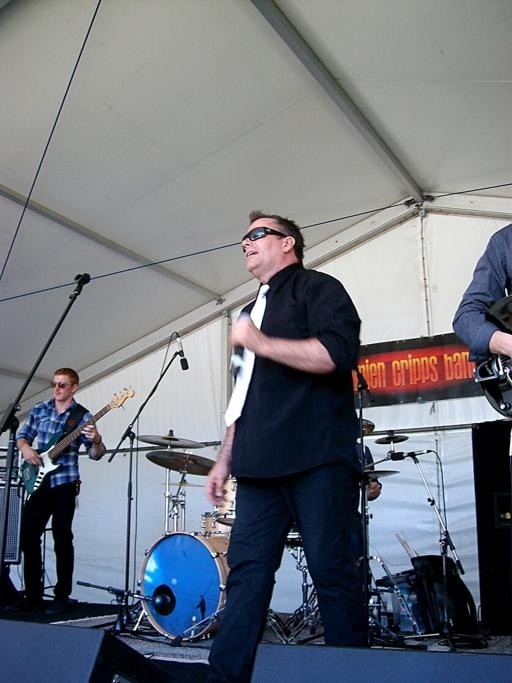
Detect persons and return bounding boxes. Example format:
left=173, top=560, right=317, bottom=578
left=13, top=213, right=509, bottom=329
left=207, top=208, right=373, bottom=682
left=14, top=365, right=108, bottom=616
left=450, top=221, right=511, bottom=364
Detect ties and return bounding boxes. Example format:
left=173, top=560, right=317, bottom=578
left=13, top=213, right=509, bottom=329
left=224, top=284, right=270, bottom=427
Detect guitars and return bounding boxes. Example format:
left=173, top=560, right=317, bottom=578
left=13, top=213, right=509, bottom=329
left=472, top=294, right=511, bottom=419
left=21, top=385, right=134, bottom=493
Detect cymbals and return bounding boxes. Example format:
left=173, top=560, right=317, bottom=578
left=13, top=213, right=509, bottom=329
left=375, top=436, right=408, bottom=444
left=357, top=418, right=375, bottom=436
left=146, top=451, right=216, bottom=476
left=361, top=468, right=398, bottom=478
left=135, top=434, right=205, bottom=449
left=140, top=530, right=231, bottom=643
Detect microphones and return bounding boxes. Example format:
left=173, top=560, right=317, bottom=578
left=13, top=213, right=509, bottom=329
left=390, top=449, right=428, bottom=462
left=176, top=334, right=189, bottom=370
left=229, top=312, right=250, bottom=376
left=68, top=273, right=90, bottom=299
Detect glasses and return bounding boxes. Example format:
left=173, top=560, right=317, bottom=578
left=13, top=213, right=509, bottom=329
left=241, top=227, right=288, bottom=253
left=49, top=382, right=77, bottom=389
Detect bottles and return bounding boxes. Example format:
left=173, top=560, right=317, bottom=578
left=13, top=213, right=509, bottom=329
left=379, top=590, right=387, bottom=628
left=401, top=592, right=421, bottom=633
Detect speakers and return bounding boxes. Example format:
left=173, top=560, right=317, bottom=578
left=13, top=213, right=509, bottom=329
left=1, top=619, right=174, bottom=683
left=471, top=422, right=512, bottom=635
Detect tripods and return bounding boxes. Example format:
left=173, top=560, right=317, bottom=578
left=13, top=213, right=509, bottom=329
left=91, top=353, right=399, bottom=647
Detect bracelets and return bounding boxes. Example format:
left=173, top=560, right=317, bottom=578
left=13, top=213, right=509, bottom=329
left=94, top=436, right=102, bottom=445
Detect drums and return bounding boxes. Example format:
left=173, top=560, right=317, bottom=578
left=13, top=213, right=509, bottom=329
left=201, top=511, right=236, bottom=534
left=285, top=521, right=302, bottom=547
left=212, top=473, right=238, bottom=526
